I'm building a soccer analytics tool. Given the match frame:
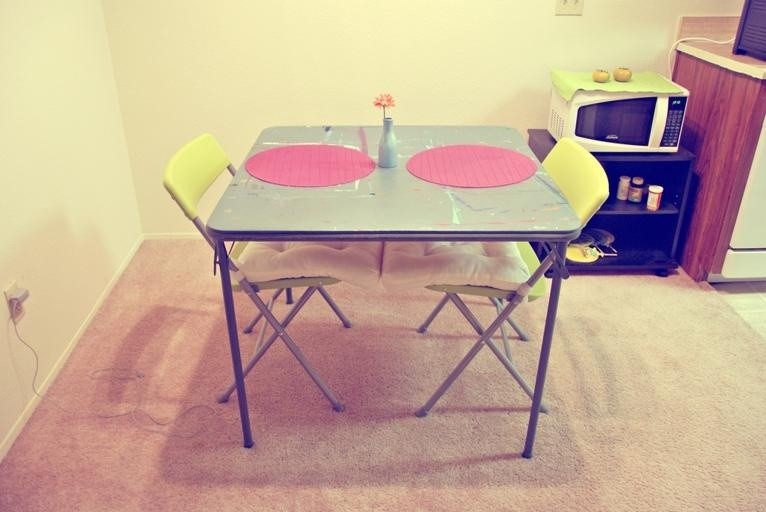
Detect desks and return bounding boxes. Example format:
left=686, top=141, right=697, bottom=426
left=206, top=125, right=583, bottom=458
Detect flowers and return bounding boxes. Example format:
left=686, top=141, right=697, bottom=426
left=374, top=92, right=392, bottom=118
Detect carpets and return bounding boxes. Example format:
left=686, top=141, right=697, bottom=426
left=0, top=240, right=766, bottom=511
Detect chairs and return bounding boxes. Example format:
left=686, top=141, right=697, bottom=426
left=382, top=136, right=609, bottom=417
left=162, top=133, right=382, bottom=412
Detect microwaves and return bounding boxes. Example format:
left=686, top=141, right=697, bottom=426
left=548, top=71, right=689, bottom=158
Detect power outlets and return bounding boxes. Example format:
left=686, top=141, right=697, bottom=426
left=4, top=282, right=27, bottom=321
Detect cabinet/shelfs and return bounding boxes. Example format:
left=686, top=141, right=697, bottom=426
left=526, top=129, right=695, bottom=277
left=673, top=39, right=766, bottom=282
